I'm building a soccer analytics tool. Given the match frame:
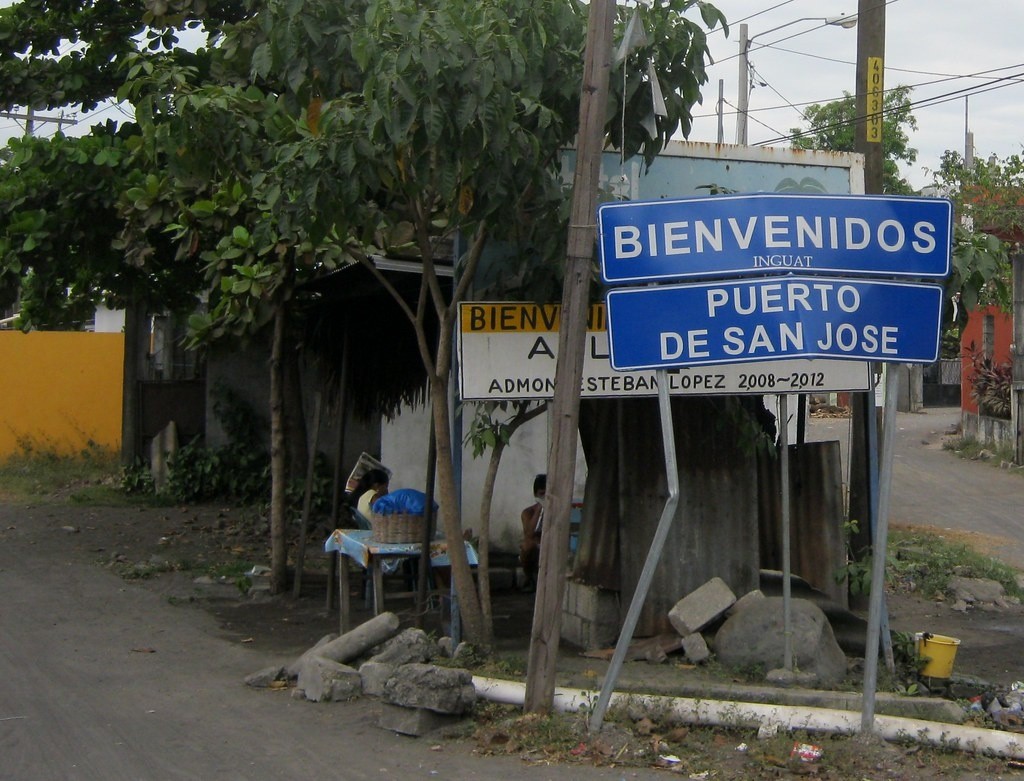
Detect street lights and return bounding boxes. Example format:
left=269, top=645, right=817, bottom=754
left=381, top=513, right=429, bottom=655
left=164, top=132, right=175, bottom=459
left=735, top=14, right=859, bottom=149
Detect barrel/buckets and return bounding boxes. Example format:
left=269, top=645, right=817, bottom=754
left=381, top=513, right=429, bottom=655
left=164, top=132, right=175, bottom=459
left=917, top=632, right=961, bottom=678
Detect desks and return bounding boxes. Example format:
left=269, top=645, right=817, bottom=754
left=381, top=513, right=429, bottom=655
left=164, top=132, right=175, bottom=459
left=323, top=529, right=478, bottom=636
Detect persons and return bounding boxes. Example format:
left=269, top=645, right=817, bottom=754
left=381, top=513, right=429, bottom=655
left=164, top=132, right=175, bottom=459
left=520, top=474, right=548, bottom=597
left=338, top=469, right=390, bottom=530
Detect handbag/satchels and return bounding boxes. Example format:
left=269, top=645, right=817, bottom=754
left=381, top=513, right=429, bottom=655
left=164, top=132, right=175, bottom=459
left=375, top=488, right=440, bottom=515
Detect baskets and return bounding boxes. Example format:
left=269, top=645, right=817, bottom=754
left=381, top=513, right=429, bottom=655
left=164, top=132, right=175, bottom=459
left=370, top=509, right=439, bottom=544
